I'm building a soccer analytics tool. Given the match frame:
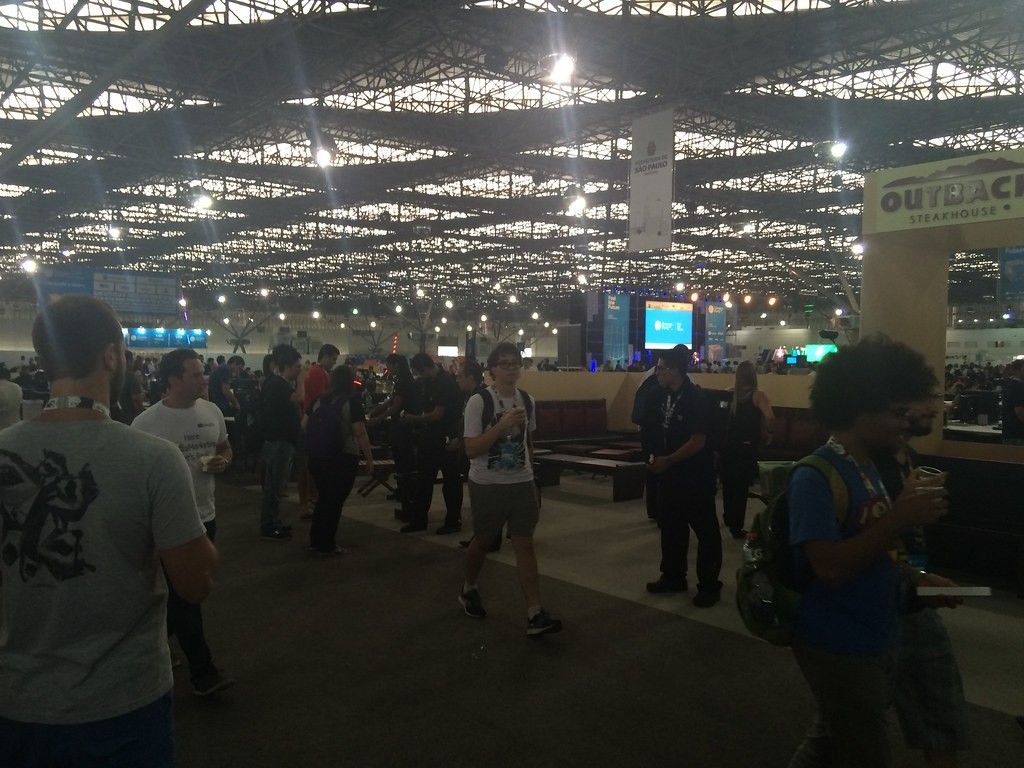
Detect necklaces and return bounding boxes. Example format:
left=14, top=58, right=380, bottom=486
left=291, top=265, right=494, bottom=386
left=46, top=396, right=111, bottom=417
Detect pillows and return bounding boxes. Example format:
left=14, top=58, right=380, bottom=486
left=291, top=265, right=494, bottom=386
left=759, top=414, right=787, bottom=449
left=536, top=408, right=562, bottom=433
left=561, top=409, right=585, bottom=433
left=583, top=408, right=608, bottom=434
left=789, top=419, right=822, bottom=450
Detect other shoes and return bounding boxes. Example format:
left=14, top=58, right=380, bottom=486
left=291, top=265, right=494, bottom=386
left=309, top=544, right=320, bottom=550
left=320, top=545, right=353, bottom=557
left=437, top=525, right=461, bottom=534
left=400, top=526, right=426, bottom=532
left=260, top=526, right=290, bottom=541
left=734, top=530, right=749, bottom=539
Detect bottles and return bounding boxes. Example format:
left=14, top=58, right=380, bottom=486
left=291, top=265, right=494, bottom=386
left=736, top=559, right=787, bottom=624
left=742, top=532, right=764, bottom=563
left=500, top=435, right=515, bottom=465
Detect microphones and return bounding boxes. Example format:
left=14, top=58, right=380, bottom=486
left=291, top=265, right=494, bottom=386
left=819, top=330, right=838, bottom=340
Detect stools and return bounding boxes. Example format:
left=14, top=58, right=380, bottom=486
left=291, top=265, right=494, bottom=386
left=356, top=459, right=397, bottom=498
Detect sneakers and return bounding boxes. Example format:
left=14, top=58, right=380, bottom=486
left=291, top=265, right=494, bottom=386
left=459, top=586, right=486, bottom=617
left=694, top=588, right=722, bottom=606
left=647, top=571, right=688, bottom=593
left=528, top=607, right=561, bottom=635
left=194, top=669, right=236, bottom=695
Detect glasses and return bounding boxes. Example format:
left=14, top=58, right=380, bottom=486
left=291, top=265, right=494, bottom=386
left=886, top=407, right=913, bottom=418
left=497, top=359, right=523, bottom=369
left=656, top=366, right=673, bottom=372
left=920, top=393, right=943, bottom=403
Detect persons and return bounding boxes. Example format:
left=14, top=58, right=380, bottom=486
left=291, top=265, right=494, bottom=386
left=0, top=294, right=1024, bottom=768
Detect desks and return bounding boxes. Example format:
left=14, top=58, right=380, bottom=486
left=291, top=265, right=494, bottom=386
left=533, top=442, right=720, bottom=502
left=954, top=391, right=1003, bottom=427
left=609, top=430, right=643, bottom=441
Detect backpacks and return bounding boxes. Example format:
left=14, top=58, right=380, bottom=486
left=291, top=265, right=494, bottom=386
left=632, top=374, right=658, bottom=425
left=233, top=399, right=265, bottom=452
left=735, top=454, right=850, bottom=646
left=304, top=390, right=350, bottom=460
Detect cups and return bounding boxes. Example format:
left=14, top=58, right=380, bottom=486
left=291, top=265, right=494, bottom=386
left=513, top=407, right=525, bottom=425
left=916, top=486, right=945, bottom=512
left=915, top=466, right=943, bottom=479
left=201, top=456, right=214, bottom=471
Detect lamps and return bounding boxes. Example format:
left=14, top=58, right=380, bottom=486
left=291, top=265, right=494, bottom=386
left=818, top=329, right=840, bottom=352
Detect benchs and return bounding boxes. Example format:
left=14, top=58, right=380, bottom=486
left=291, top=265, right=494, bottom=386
left=532, top=399, right=623, bottom=453
left=758, top=406, right=829, bottom=461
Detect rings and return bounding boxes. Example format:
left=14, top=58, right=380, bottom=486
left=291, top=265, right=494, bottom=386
left=219, top=465, right=221, bottom=469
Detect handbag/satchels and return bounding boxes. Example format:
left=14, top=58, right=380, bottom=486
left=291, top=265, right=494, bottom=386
left=719, top=448, right=731, bottom=476
